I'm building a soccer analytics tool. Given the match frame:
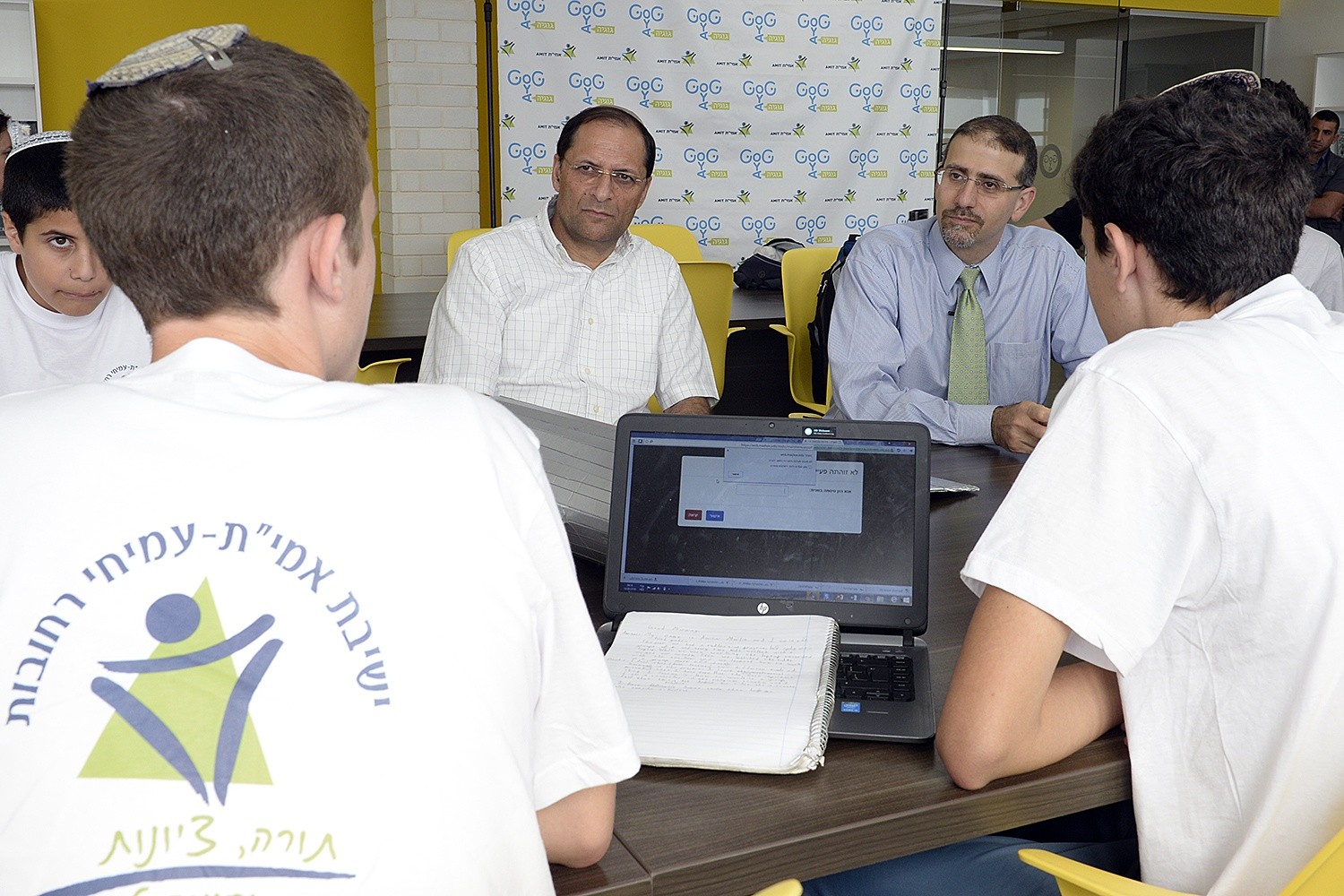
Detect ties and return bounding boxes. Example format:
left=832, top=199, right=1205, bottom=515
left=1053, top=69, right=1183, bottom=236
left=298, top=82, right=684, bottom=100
left=947, top=268, right=988, bottom=406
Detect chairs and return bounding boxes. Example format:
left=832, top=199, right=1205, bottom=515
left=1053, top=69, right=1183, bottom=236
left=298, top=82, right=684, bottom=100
left=358, top=218, right=852, bottom=421
left=1021, top=829, right=1344, bottom=896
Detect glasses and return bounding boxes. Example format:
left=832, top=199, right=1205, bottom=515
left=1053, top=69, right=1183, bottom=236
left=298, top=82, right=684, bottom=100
left=561, top=154, right=648, bottom=189
left=934, top=166, right=1027, bottom=199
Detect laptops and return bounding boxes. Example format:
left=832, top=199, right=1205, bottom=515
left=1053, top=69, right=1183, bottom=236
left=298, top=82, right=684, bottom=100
left=598, top=414, right=937, bottom=743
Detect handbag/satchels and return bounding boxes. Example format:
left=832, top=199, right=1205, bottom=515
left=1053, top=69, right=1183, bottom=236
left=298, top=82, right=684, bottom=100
left=733, top=238, right=806, bottom=290
left=715, top=324, right=811, bottom=417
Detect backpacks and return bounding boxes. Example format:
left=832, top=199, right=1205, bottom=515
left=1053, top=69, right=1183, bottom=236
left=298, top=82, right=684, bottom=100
left=807, top=234, right=862, bottom=405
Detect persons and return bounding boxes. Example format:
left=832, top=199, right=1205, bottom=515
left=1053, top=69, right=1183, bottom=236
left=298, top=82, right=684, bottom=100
left=0, top=24, right=640, bottom=895
left=1, top=111, right=154, bottom=399
left=804, top=78, right=1343, bottom=896
left=416, top=106, right=720, bottom=418
left=820, top=115, right=1109, bottom=454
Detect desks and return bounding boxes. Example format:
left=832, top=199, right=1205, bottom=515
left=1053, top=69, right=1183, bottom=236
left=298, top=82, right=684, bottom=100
left=364, top=291, right=438, bottom=362
left=550, top=431, right=1134, bottom=896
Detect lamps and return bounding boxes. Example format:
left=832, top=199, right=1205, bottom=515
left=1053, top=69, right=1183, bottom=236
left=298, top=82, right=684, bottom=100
left=946, top=35, right=1064, bottom=55
